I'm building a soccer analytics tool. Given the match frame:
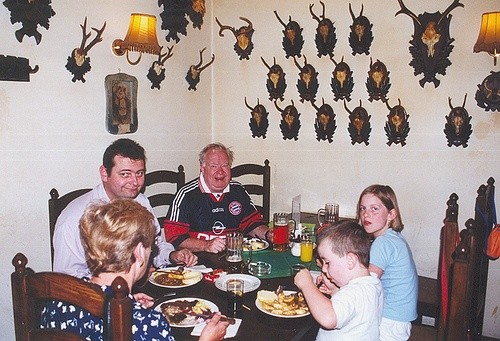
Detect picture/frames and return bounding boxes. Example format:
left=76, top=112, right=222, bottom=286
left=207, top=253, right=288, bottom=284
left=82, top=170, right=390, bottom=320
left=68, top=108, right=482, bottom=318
left=104, top=73, right=138, bottom=134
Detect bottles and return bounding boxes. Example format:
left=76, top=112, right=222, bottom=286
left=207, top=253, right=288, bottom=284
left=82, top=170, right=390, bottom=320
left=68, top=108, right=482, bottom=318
left=291, top=238, right=301, bottom=257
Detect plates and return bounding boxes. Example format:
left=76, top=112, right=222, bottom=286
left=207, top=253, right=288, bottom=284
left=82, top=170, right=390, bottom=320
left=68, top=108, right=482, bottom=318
left=215, top=273, right=262, bottom=294
left=228, top=237, right=269, bottom=252
left=154, top=297, right=220, bottom=328
left=148, top=268, right=203, bottom=288
left=255, top=290, right=311, bottom=318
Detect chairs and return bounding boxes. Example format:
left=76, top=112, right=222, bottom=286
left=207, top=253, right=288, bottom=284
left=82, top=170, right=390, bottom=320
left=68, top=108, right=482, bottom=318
left=230, top=159, right=270, bottom=225
left=137, top=165, right=185, bottom=233
left=407, top=176, right=495, bottom=341
left=49, top=188, right=96, bottom=265
left=11, top=253, right=133, bottom=341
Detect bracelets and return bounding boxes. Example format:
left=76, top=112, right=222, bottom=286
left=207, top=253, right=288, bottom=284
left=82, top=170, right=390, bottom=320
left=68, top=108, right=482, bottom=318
left=265, top=230, right=269, bottom=243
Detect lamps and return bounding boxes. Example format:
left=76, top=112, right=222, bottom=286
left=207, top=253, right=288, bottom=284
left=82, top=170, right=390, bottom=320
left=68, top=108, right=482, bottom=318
left=473, top=12, right=500, bottom=66
left=112, top=12, right=161, bottom=66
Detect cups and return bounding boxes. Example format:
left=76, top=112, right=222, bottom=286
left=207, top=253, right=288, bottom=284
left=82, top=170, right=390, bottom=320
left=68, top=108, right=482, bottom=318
left=300, top=239, right=313, bottom=263
left=227, top=279, right=244, bottom=317
left=318, top=202, right=339, bottom=227
left=226, top=232, right=252, bottom=272
left=273, top=212, right=296, bottom=252
left=290, top=264, right=305, bottom=279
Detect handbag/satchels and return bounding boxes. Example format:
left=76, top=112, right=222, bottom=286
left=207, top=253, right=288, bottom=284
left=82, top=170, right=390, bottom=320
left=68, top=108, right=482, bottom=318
left=486, top=224, right=500, bottom=261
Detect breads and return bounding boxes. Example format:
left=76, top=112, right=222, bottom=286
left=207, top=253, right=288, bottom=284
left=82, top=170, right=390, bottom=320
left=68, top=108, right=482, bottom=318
left=182, top=271, right=202, bottom=284
left=250, top=241, right=264, bottom=249
left=257, top=290, right=278, bottom=303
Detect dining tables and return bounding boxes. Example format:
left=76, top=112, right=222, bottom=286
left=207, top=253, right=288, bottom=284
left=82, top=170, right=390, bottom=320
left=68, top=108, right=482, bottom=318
left=126, top=210, right=359, bottom=341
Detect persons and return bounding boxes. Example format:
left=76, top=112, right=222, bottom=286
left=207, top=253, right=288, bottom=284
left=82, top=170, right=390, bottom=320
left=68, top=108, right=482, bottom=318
left=296, top=185, right=418, bottom=341
left=160, top=144, right=276, bottom=252
left=32, top=199, right=228, bottom=341
left=52, top=139, right=199, bottom=280
